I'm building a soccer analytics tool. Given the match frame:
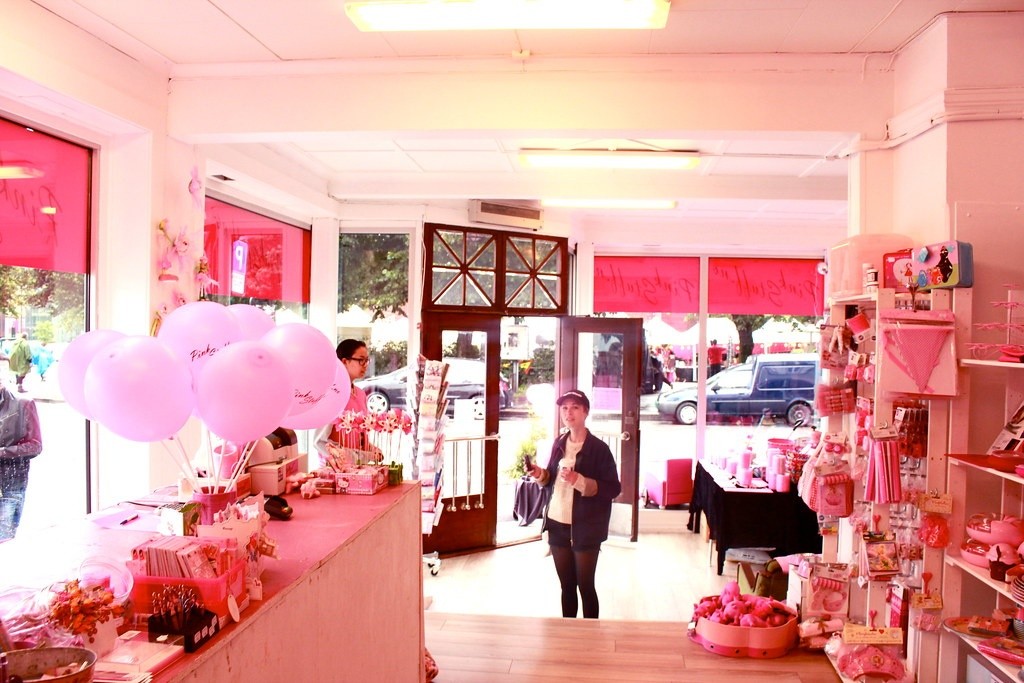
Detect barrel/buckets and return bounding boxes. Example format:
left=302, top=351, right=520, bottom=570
left=767, top=439, right=794, bottom=455
left=192, top=486, right=237, bottom=526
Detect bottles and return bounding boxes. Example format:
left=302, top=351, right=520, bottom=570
left=177, top=464, right=197, bottom=502
left=1014, top=604, right=1024, bottom=639
left=824, top=443, right=852, bottom=464
left=862, top=263, right=879, bottom=294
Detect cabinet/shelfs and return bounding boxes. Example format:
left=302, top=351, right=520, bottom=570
left=964, top=290, right=1024, bottom=363
left=148, top=480, right=426, bottom=683
left=829, top=287, right=952, bottom=683
left=942, top=288, right=1024, bottom=683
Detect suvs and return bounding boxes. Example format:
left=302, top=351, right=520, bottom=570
left=354, top=357, right=512, bottom=420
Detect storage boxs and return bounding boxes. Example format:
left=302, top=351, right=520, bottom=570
left=124, top=557, right=250, bottom=631
left=314, top=479, right=336, bottom=494
left=310, top=465, right=335, bottom=479
left=247, top=453, right=309, bottom=495
left=388, top=465, right=404, bottom=486
left=334, top=465, right=390, bottom=495
left=882, top=251, right=929, bottom=294
left=911, top=240, right=974, bottom=289
left=159, top=500, right=203, bottom=538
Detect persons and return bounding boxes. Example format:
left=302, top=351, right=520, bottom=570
left=313, top=339, right=384, bottom=465
left=31, top=342, right=54, bottom=380
left=8, top=332, right=33, bottom=392
left=524, top=389, right=622, bottom=618
left=654, top=344, right=676, bottom=391
left=0, top=379, right=42, bottom=541
left=708, top=339, right=726, bottom=376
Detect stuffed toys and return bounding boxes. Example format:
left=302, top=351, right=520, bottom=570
left=695, top=581, right=794, bottom=628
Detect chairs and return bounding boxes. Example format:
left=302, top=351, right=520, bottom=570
left=735, top=552, right=823, bottom=601
left=640, top=458, right=695, bottom=511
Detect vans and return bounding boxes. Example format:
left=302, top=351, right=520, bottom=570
left=655, top=353, right=819, bottom=427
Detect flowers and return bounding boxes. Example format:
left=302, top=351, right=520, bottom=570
left=41, top=576, right=125, bottom=640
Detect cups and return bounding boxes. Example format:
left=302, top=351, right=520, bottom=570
left=848, top=313, right=869, bottom=335
left=740, top=470, right=752, bottom=485
left=729, top=461, right=738, bottom=475
left=766, top=448, right=786, bottom=475
left=214, top=444, right=238, bottom=479
left=766, top=470, right=790, bottom=492
left=718, top=456, right=726, bottom=470
left=559, top=458, right=574, bottom=482
left=742, top=453, right=751, bottom=469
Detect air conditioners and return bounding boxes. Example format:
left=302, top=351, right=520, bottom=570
left=467, top=200, right=545, bottom=232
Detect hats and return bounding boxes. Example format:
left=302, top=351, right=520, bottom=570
left=557, top=390, right=589, bottom=412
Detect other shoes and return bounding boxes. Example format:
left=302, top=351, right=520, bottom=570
left=670, top=383, right=674, bottom=389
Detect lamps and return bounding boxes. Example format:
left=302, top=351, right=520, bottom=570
left=341, top=0, right=673, bottom=35
left=516, top=148, right=700, bottom=171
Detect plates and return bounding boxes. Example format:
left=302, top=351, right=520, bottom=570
left=1010, top=574, right=1024, bottom=604
left=944, top=616, right=1013, bottom=641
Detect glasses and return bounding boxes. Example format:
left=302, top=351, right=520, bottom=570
left=344, top=357, right=371, bottom=366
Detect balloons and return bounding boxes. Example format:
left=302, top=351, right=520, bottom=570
left=56, top=301, right=351, bottom=442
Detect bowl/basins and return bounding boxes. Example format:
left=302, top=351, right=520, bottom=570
left=0, top=647, right=97, bottom=683
left=989, top=451, right=1024, bottom=472
left=74, top=556, right=133, bottom=607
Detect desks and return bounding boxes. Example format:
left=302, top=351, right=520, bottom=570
left=513, top=477, right=546, bottom=527
left=421, top=610, right=842, bottom=683
left=686, top=459, right=824, bottom=575
